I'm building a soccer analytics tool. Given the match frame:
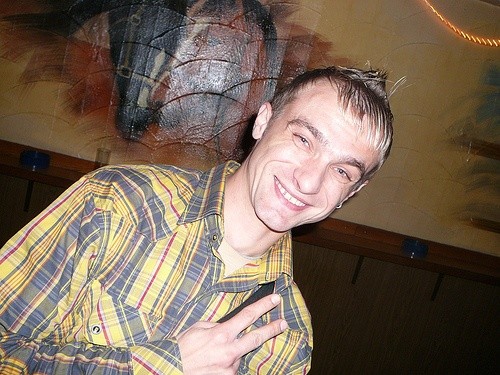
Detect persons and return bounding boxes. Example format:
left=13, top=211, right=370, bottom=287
left=0, top=66, right=395, bottom=375
